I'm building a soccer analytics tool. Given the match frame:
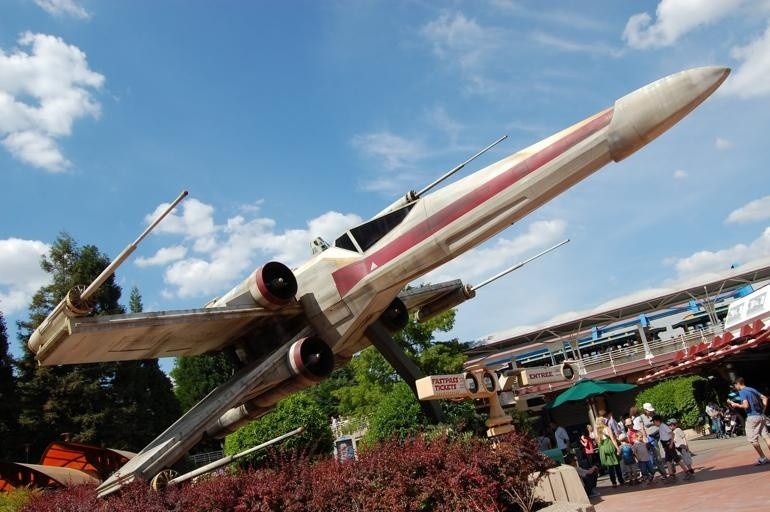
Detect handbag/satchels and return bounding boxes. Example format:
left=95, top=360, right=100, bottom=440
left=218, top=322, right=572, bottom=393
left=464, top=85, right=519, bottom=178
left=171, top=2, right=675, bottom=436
left=752, top=392, right=764, bottom=412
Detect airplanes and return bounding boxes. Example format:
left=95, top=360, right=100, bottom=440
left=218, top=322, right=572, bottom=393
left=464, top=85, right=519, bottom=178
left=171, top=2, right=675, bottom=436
left=11, top=62, right=733, bottom=501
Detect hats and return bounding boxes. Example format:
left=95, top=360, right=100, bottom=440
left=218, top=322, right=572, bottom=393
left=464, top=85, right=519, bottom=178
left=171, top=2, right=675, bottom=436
left=624, top=418, right=632, bottom=426
left=650, top=415, right=660, bottom=422
left=643, top=403, right=655, bottom=412
left=619, top=434, right=627, bottom=441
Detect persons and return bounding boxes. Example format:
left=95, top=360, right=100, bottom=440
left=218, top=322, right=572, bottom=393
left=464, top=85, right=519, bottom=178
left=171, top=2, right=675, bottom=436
left=545, top=340, right=639, bottom=368
left=337, top=414, right=345, bottom=437
left=727, top=377, right=770, bottom=465
left=534, top=401, right=738, bottom=497
left=329, top=414, right=338, bottom=439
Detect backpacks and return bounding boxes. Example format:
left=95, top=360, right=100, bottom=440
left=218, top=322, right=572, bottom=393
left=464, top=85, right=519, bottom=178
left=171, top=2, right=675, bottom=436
left=621, top=445, right=633, bottom=465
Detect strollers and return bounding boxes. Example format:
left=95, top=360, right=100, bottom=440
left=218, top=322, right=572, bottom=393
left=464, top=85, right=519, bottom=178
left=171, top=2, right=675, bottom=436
left=720, top=411, right=738, bottom=440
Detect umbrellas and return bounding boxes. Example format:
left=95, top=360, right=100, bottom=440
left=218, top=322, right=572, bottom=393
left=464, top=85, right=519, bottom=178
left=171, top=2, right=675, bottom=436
left=542, top=377, right=638, bottom=422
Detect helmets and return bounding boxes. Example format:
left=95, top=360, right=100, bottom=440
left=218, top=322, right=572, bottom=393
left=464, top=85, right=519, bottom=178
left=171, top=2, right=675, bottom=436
left=666, top=418, right=677, bottom=424
